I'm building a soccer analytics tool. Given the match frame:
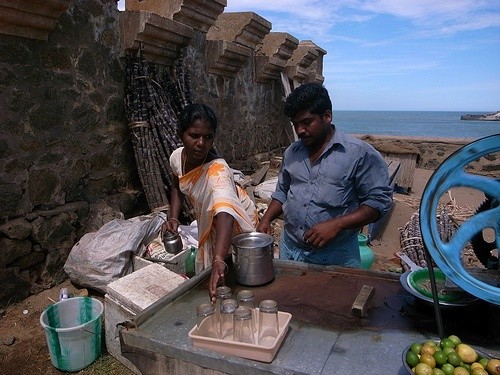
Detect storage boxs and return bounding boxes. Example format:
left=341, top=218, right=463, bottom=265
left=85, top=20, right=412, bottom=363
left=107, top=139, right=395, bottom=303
left=104, top=243, right=191, bottom=375
left=188, top=305, right=293, bottom=364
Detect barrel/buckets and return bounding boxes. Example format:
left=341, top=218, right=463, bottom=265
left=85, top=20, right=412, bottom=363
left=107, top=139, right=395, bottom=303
left=40, top=296, right=104, bottom=372
left=230, top=231, right=276, bottom=286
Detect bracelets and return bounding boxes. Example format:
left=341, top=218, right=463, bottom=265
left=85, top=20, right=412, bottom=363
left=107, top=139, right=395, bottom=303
left=167, top=217, right=181, bottom=224
left=211, top=260, right=229, bottom=274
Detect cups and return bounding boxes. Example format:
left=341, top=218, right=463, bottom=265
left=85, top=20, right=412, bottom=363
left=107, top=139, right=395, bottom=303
left=195, top=303, right=218, bottom=339
left=212, top=286, right=232, bottom=318
left=231, top=306, right=255, bottom=345
left=237, top=290, right=256, bottom=328
left=218, top=298, right=238, bottom=341
left=257, top=299, right=279, bottom=348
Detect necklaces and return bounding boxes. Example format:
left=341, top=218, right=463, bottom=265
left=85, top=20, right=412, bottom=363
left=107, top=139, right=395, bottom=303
left=183, top=153, right=208, bottom=176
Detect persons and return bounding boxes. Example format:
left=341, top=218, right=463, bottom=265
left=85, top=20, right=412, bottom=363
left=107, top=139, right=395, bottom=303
left=255, top=82, right=394, bottom=270
left=160, top=102, right=259, bottom=303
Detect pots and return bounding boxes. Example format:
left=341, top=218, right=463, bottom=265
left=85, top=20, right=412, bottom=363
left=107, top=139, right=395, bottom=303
left=394, top=252, right=481, bottom=318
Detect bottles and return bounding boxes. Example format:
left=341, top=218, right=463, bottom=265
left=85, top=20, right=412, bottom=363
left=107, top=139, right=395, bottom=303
left=185, top=247, right=197, bottom=279
left=163, top=231, right=183, bottom=253
left=59, top=288, right=70, bottom=301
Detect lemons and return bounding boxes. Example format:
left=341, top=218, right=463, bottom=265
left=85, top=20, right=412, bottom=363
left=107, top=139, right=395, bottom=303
left=406, top=334, right=500, bottom=375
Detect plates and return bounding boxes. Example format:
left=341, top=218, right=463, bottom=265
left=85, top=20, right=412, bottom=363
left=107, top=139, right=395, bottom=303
left=401, top=338, right=497, bottom=374
